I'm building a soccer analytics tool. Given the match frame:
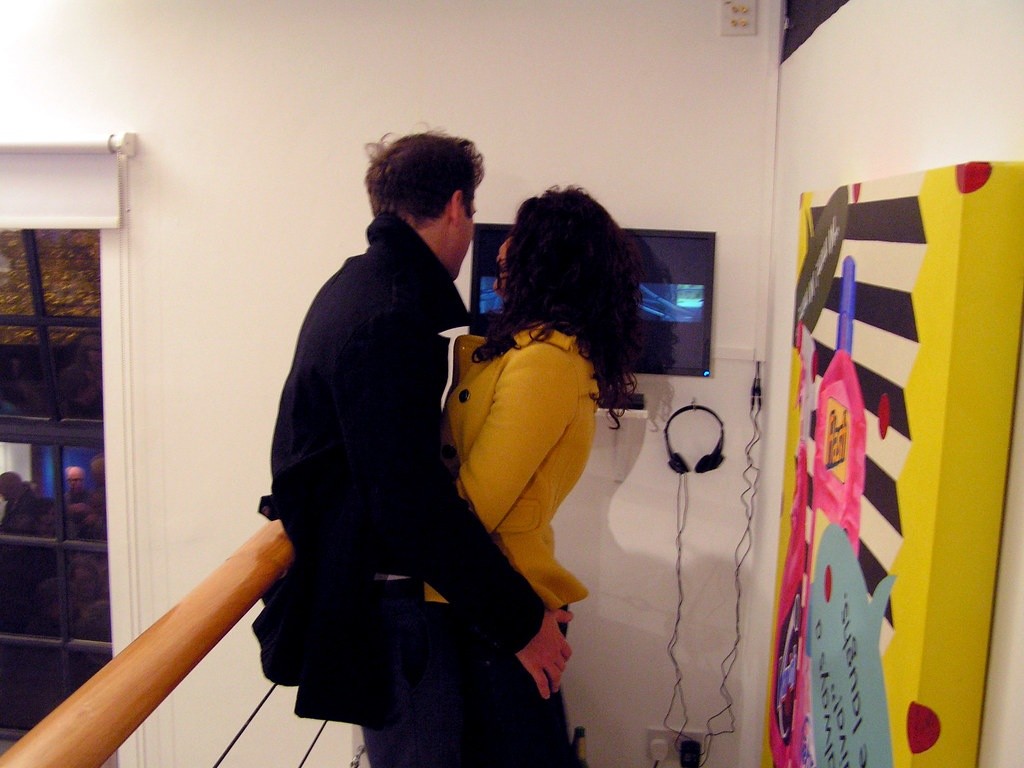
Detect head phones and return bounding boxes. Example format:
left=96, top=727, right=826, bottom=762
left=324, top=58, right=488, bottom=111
left=664, top=405, right=725, bottom=474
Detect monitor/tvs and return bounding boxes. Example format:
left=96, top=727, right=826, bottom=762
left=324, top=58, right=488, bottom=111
left=470, top=223, right=716, bottom=378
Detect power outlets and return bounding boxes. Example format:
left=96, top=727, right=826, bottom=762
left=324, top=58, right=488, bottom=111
left=645, top=726, right=703, bottom=761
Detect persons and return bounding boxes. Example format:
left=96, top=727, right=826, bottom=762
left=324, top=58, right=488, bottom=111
left=13, top=497, right=106, bottom=623
left=0, top=472, right=22, bottom=513
left=65, top=467, right=88, bottom=501
left=90, top=455, right=105, bottom=489
left=252, top=132, right=574, bottom=767
left=425, top=190, right=638, bottom=767
